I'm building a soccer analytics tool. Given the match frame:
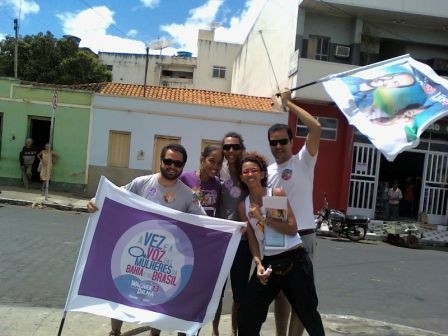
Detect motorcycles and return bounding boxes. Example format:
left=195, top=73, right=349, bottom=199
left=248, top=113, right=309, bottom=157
left=314, top=192, right=370, bottom=241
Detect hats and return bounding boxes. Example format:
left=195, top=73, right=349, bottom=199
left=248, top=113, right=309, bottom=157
left=405, top=177, right=412, bottom=181
left=392, top=180, right=400, bottom=184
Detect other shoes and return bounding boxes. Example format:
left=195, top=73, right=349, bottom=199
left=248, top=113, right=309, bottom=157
left=109, top=330, right=120, bottom=336
left=149, top=331, right=161, bottom=336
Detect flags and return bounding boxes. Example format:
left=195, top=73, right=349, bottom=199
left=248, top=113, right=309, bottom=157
left=65, top=175, right=248, bottom=336
left=322, top=52, right=448, bottom=162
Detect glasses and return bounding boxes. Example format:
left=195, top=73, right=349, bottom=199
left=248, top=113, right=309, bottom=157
left=269, top=138, right=290, bottom=146
left=223, top=144, right=241, bottom=150
left=162, top=158, right=183, bottom=167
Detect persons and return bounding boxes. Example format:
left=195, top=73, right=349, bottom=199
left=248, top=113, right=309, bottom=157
left=380, top=177, right=418, bottom=221
left=338, top=71, right=431, bottom=149
left=20, top=138, right=60, bottom=191
left=86, top=144, right=248, bottom=335
left=236, top=154, right=326, bottom=336
left=176, top=87, right=322, bottom=336
